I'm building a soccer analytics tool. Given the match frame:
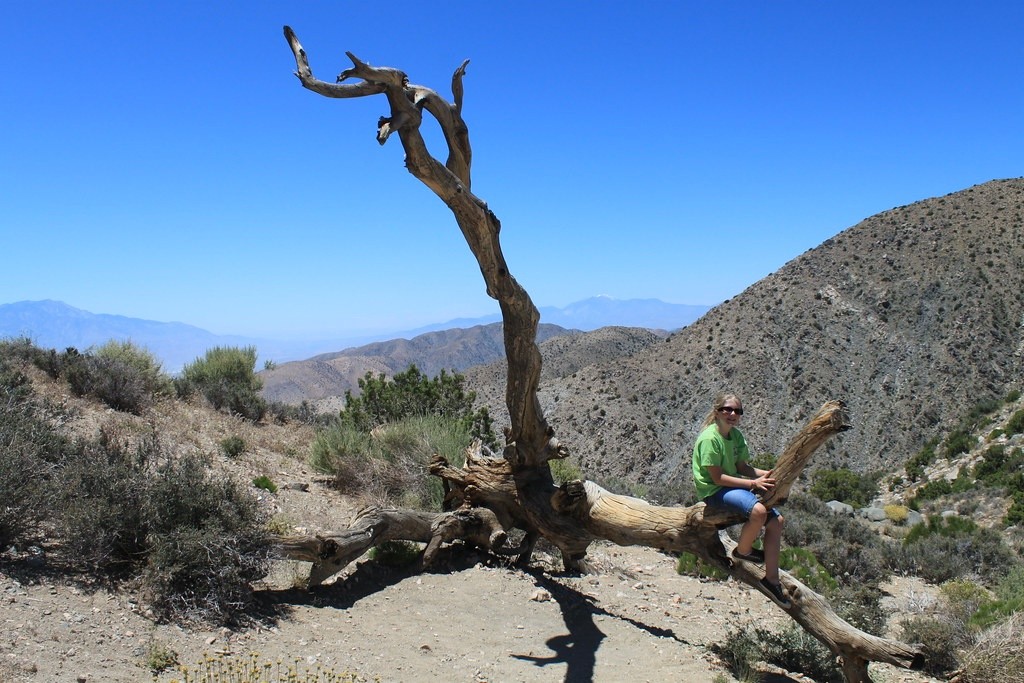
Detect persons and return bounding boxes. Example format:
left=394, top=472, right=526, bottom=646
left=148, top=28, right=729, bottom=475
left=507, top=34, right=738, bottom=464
left=692, top=394, right=792, bottom=609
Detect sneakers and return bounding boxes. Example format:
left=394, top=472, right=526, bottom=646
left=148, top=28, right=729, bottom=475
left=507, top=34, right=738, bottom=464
left=732, top=547, right=765, bottom=563
left=757, top=576, right=791, bottom=609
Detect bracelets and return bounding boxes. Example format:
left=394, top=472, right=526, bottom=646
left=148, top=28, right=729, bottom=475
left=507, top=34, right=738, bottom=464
left=751, top=479, right=757, bottom=489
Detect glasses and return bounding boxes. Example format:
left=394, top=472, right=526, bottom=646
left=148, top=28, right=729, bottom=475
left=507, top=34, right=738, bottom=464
left=718, top=406, right=743, bottom=415
